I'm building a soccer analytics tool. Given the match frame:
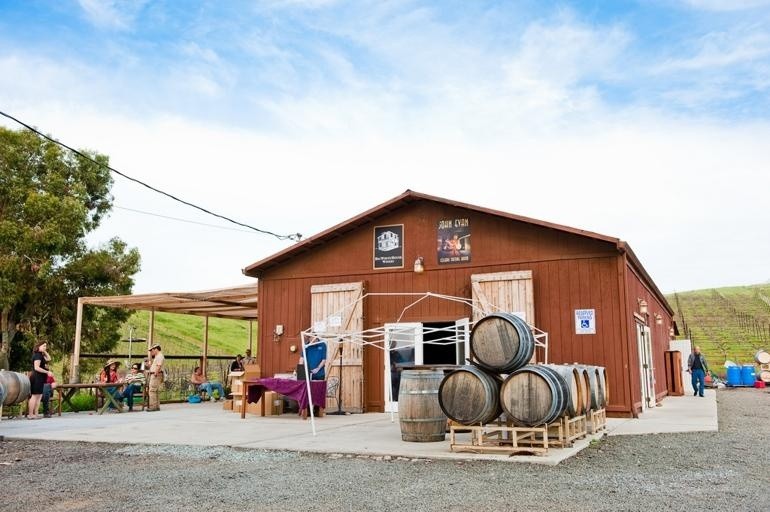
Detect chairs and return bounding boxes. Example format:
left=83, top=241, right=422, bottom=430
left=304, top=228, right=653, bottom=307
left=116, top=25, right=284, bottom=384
left=94, top=387, right=103, bottom=409
left=126, top=370, right=164, bottom=410
left=325, top=376, right=339, bottom=408
left=193, top=384, right=206, bottom=400
left=25, top=385, right=61, bottom=416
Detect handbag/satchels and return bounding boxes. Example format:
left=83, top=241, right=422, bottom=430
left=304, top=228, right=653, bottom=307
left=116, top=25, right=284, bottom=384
left=163, top=367, right=168, bottom=383
left=188, top=395, right=201, bottom=403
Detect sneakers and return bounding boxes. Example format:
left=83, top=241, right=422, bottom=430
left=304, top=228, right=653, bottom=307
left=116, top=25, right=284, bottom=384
left=146, top=408, right=160, bottom=411
left=210, top=397, right=227, bottom=402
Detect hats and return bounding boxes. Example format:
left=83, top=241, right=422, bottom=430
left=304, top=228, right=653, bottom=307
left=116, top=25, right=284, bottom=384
left=246, top=349, right=251, bottom=353
left=148, top=343, right=160, bottom=351
left=105, top=359, right=120, bottom=367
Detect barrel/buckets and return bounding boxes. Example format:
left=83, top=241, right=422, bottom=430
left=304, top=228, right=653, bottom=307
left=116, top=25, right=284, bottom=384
left=0, top=370, right=31, bottom=403
left=470, top=310, right=536, bottom=375
left=437, top=364, right=503, bottom=426
left=541, top=360, right=610, bottom=418
left=397, top=369, right=446, bottom=442
left=500, top=364, right=570, bottom=426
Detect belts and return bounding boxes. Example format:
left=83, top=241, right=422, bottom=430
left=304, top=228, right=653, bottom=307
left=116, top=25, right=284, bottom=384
left=148, top=371, right=163, bottom=375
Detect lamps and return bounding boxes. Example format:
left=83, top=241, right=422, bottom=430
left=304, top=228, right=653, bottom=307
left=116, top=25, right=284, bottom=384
left=413, top=256, right=424, bottom=274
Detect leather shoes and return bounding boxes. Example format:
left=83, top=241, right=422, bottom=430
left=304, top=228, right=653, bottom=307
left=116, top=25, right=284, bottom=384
left=694, top=388, right=704, bottom=397
left=27, top=414, right=53, bottom=420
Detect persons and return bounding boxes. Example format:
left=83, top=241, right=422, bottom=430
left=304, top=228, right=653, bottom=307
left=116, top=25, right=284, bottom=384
left=231, top=349, right=255, bottom=371
left=299, top=332, right=326, bottom=420
left=688, top=346, right=709, bottom=397
left=443, top=234, right=470, bottom=257
left=100, top=343, right=165, bottom=412
left=27, top=341, right=55, bottom=420
left=581, top=320, right=588, bottom=328
left=191, top=367, right=226, bottom=402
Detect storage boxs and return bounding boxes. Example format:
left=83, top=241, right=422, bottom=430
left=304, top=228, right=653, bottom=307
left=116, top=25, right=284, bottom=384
left=223, top=363, right=284, bottom=416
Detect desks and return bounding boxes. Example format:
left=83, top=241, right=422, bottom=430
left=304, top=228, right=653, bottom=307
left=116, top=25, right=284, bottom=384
left=238, top=373, right=325, bottom=419
left=58, top=383, right=124, bottom=415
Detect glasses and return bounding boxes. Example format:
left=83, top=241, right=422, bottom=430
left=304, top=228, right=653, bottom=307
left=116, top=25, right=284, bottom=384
left=132, top=367, right=138, bottom=370
